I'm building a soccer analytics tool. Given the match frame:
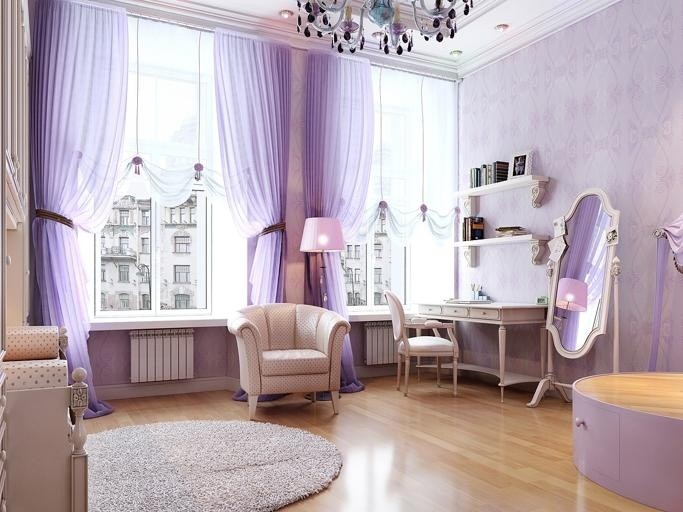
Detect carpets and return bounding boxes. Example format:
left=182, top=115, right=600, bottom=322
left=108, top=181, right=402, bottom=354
left=83, top=419, right=343, bottom=511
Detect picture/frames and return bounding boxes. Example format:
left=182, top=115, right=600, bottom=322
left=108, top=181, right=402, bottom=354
left=506, top=151, right=533, bottom=180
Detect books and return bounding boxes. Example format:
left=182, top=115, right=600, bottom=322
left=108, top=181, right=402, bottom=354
left=461, top=160, right=510, bottom=242
left=493, top=225, right=529, bottom=237
left=453, top=300, right=491, bottom=305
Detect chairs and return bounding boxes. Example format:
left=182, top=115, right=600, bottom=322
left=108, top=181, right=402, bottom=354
left=226, top=302, right=351, bottom=420
left=385, top=290, right=459, bottom=397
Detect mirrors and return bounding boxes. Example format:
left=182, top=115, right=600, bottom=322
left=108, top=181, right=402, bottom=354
left=525, top=189, right=620, bottom=408
left=555, top=277, right=588, bottom=329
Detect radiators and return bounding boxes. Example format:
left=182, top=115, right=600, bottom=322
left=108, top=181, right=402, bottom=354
left=362, top=322, right=410, bottom=366
left=127, top=329, right=196, bottom=385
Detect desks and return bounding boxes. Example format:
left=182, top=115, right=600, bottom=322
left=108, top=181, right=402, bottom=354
left=414, top=302, right=548, bottom=403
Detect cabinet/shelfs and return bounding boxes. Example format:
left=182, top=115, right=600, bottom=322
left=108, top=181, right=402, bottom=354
left=449, top=176, right=550, bottom=266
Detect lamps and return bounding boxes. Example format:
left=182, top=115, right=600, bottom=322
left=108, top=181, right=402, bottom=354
left=299, top=217, right=345, bottom=307
left=295, top=0, right=474, bottom=55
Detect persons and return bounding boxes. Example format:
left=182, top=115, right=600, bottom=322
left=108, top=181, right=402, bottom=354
left=514, top=157, right=524, bottom=175
